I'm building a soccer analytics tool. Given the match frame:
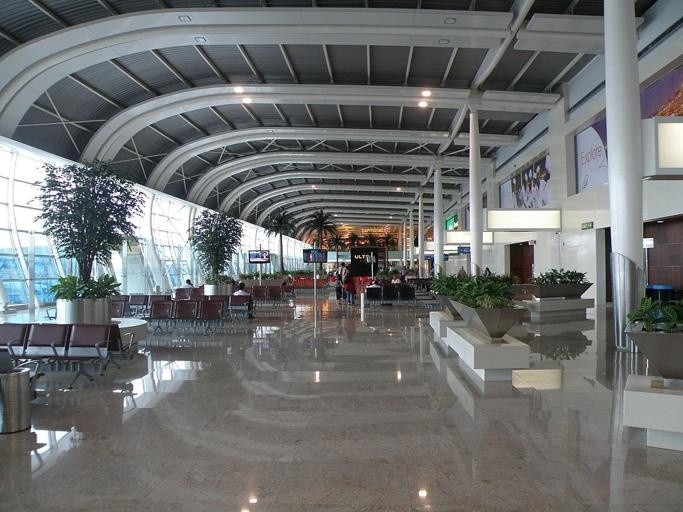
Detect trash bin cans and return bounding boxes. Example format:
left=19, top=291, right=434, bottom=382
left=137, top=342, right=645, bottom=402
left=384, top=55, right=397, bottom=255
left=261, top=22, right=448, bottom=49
left=0, top=367, right=31, bottom=434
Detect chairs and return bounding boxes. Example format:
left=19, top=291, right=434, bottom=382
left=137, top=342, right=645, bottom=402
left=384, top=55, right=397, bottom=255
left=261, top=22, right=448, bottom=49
left=0, top=278, right=295, bottom=386
left=366, top=280, right=417, bottom=306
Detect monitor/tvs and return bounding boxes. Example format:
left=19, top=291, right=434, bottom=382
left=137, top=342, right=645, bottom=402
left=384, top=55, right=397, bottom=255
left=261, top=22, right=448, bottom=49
left=248, top=249, right=271, bottom=264
left=303, top=249, right=328, bottom=263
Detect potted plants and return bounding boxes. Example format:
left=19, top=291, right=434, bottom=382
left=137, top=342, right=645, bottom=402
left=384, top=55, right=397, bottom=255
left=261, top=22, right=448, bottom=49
left=623, top=296, right=683, bottom=380
left=427, top=269, right=595, bottom=344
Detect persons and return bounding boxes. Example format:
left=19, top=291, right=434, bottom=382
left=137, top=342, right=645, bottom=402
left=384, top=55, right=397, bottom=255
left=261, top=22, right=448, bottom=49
left=456, top=266, right=466, bottom=277
left=485, top=267, right=490, bottom=276
left=333, top=261, right=356, bottom=306
left=232, top=282, right=255, bottom=319
left=429, top=266, right=434, bottom=277
left=389, top=275, right=401, bottom=284
left=510, top=167, right=551, bottom=210
left=260, top=252, right=269, bottom=258
left=184, top=279, right=194, bottom=288
left=397, top=264, right=410, bottom=283
